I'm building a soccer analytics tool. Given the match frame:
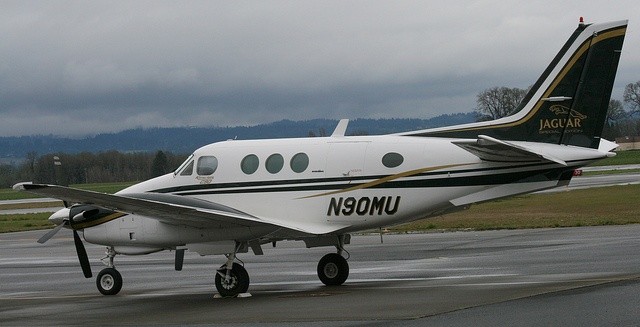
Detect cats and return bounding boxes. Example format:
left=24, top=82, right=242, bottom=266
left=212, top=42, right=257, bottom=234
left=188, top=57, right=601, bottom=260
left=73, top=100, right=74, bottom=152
left=13, top=16, right=629, bottom=298
